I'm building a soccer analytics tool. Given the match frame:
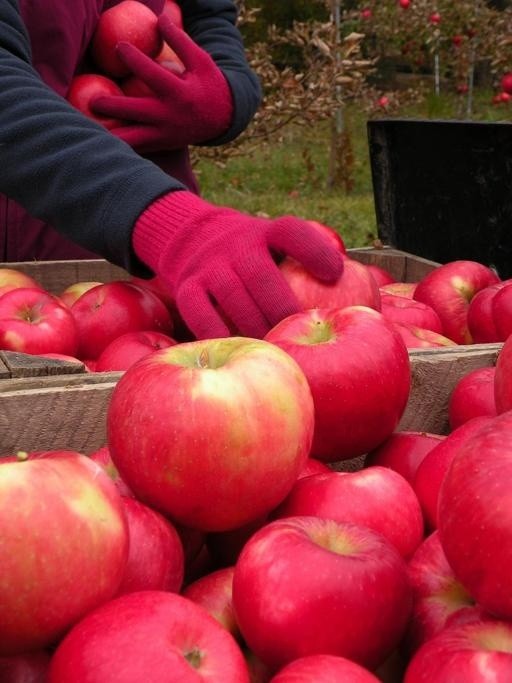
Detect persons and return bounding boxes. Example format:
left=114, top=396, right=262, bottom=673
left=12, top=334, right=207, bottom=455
left=0, top=1, right=344, bottom=337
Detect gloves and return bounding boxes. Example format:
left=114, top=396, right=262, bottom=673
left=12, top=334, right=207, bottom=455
left=131, top=190, right=344, bottom=340
left=95, top=15, right=233, bottom=152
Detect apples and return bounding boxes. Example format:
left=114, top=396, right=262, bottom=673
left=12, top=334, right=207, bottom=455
left=451, top=34, right=462, bottom=45
left=455, top=86, right=468, bottom=93
left=65, top=1, right=187, bottom=130
left=400, top=1, right=409, bottom=8
left=492, top=94, right=501, bottom=105
left=499, top=92, right=511, bottom=103
left=430, top=12, right=440, bottom=24
left=0, top=219, right=512, bottom=683
left=378, top=97, right=389, bottom=106
left=362, top=8, right=371, bottom=18
left=466, top=29, right=478, bottom=38
left=501, top=73, right=512, bottom=96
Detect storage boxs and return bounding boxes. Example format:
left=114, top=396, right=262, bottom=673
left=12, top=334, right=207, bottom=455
left=1, top=350, right=501, bottom=473
left=0, top=243, right=512, bottom=389
